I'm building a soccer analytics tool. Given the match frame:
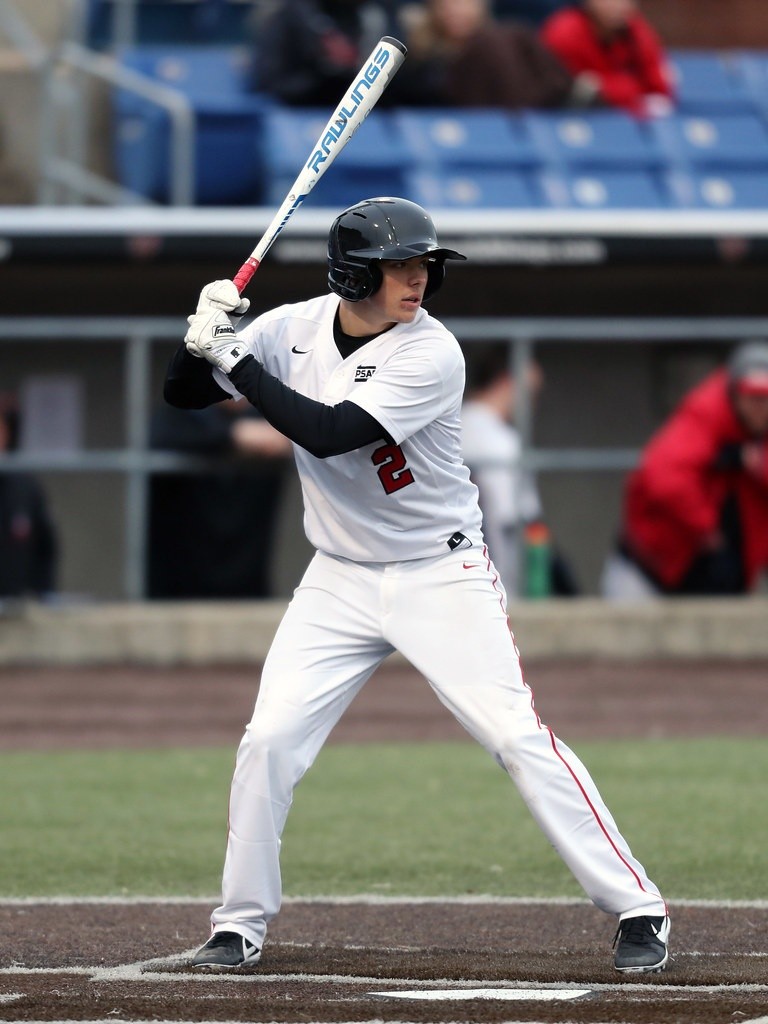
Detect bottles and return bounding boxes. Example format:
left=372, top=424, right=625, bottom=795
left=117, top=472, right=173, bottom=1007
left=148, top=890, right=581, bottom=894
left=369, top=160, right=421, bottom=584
left=522, top=520, right=551, bottom=598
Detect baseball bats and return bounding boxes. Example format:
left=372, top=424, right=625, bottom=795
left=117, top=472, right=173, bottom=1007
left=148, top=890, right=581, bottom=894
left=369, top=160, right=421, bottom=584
left=181, top=35, right=410, bottom=363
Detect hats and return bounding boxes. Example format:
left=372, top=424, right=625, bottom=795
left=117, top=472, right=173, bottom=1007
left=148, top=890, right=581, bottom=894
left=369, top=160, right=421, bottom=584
left=724, top=339, right=768, bottom=397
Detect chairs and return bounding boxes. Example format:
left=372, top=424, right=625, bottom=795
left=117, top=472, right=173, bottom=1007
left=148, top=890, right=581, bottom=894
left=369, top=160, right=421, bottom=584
left=108, top=48, right=768, bottom=212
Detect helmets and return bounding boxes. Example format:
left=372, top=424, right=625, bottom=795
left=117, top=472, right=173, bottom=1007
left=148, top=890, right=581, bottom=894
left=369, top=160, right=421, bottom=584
left=328, top=196, right=467, bottom=302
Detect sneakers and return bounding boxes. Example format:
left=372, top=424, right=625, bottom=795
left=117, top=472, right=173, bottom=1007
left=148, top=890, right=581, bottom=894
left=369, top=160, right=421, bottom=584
left=190, top=930, right=261, bottom=971
left=612, top=912, right=671, bottom=976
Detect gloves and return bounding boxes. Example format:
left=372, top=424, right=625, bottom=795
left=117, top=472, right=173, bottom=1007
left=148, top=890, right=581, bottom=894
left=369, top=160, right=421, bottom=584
left=184, top=279, right=251, bottom=375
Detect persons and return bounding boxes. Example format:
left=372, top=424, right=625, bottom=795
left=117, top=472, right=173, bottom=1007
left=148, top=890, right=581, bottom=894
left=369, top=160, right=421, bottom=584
left=608, top=340, right=768, bottom=599
left=458, top=346, right=547, bottom=596
left=536, top=2, right=680, bottom=119
left=145, top=380, right=292, bottom=599
left=163, top=195, right=672, bottom=973
left=247, top=0, right=608, bottom=113
